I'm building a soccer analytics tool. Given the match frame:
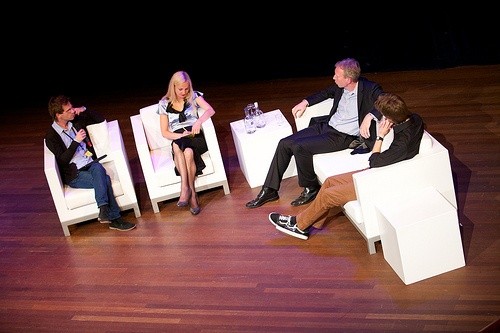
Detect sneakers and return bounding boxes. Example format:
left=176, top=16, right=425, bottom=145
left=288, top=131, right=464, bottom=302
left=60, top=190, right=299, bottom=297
left=98, top=206, right=112, bottom=224
left=269, top=212, right=310, bottom=240
left=108, top=218, right=136, bottom=231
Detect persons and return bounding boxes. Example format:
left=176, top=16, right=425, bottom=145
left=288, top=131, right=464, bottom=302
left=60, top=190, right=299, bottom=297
left=246, top=58, right=384, bottom=208
left=157, top=71, right=215, bottom=215
left=269, top=90, right=424, bottom=240
left=46, top=95, right=136, bottom=231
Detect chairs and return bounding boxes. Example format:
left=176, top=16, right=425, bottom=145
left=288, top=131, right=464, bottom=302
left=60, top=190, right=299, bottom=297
left=129, top=108, right=230, bottom=213
left=44, top=120, right=141, bottom=236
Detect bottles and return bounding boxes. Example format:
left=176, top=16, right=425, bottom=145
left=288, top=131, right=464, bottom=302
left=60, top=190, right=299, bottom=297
left=241, top=102, right=266, bottom=133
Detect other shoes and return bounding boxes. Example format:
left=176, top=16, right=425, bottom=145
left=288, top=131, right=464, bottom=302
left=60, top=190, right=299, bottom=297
left=177, top=201, right=188, bottom=207
left=190, top=208, right=200, bottom=215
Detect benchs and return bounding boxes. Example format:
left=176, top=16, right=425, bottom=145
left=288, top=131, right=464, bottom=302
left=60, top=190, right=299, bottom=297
left=294, top=98, right=458, bottom=255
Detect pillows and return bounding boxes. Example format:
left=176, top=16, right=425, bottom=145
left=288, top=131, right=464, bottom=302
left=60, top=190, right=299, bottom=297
left=86, top=119, right=112, bottom=164
left=140, top=103, right=171, bottom=150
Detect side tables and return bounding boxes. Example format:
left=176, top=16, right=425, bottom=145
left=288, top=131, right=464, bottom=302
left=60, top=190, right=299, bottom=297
left=376, top=186, right=466, bottom=285
left=229, top=109, right=298, bottom=189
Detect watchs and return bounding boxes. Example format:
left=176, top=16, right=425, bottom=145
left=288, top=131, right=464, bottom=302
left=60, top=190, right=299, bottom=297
left=376, top=136, right=383, bottom=141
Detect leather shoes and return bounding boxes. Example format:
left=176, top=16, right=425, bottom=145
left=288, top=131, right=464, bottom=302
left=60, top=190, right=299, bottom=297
left=246, top=188, right=280, bottom=208
left=291, top=185, right=322, bottom=206
